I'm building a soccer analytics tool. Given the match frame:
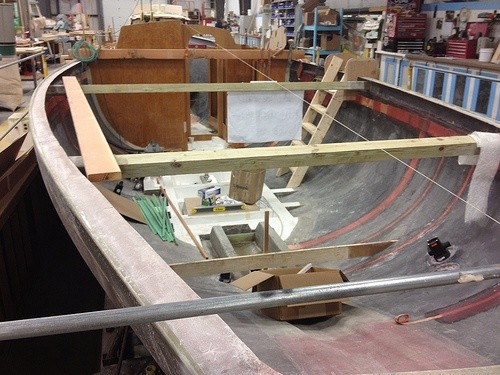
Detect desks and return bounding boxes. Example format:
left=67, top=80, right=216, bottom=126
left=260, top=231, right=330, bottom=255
left=14, top=46, right=48, bottom=89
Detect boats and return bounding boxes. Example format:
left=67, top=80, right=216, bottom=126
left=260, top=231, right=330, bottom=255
left=27, top=18, right=500, bottom=375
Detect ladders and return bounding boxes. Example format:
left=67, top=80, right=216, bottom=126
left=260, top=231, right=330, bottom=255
left=275, top=55, right=356, bottom=189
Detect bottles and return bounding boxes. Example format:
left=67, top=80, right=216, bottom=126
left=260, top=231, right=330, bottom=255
left=113, top=181, right=123, bottom=195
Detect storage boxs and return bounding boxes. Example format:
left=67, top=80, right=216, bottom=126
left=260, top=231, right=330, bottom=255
left=230, top=267, right=352, bottom=320
left=228, top=169, right=266, bottom=205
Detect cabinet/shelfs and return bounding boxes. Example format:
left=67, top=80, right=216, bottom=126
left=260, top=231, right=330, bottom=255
left=267, top=0, right=343, bottom=63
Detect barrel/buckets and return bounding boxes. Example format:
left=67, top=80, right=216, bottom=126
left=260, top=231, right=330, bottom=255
left=478, top=48, right=495, bottom=62
